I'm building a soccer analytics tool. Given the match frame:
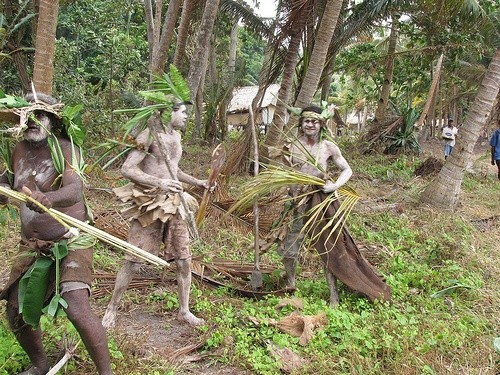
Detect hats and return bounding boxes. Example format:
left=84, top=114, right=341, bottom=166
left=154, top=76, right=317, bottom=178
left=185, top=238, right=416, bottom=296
left=0, top=102, right=64, bottom=124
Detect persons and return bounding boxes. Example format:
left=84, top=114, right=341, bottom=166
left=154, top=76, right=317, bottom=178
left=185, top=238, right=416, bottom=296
left=489, top=120, right=500, bottom=180
left=0, top=93, right=114, bottom=375
left=442, top=119, right=458, bottom=160
left=336, top=121, right=344, bottom=137
left=226, top=122, right=266, bottom=137
left=279, top=107, right=352, bottom=310
left=100, top=95, right=217, bottom=333
